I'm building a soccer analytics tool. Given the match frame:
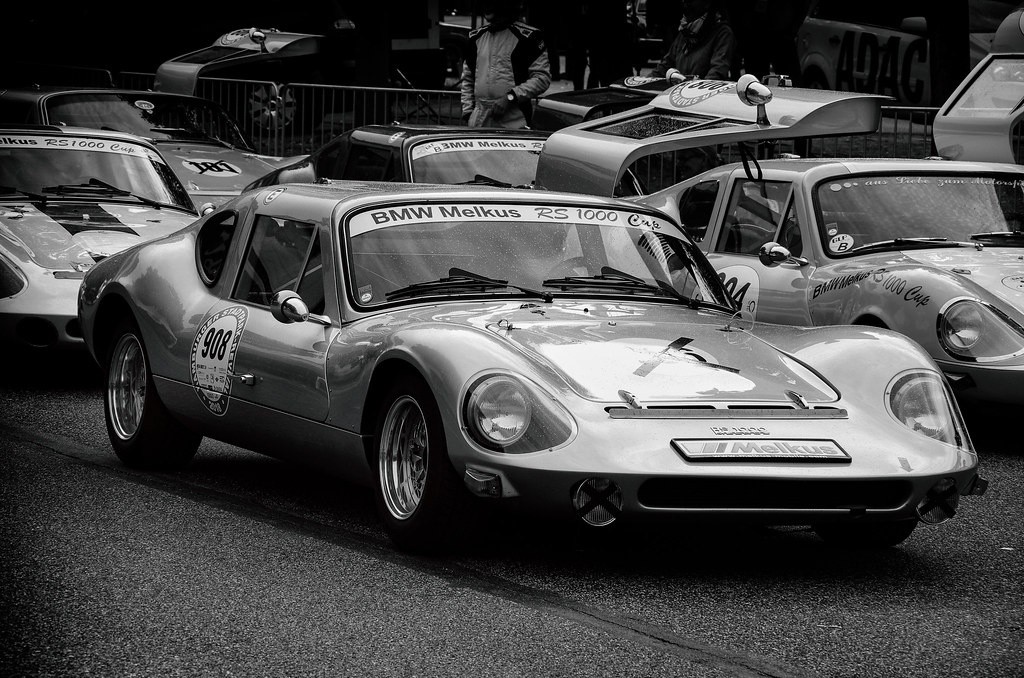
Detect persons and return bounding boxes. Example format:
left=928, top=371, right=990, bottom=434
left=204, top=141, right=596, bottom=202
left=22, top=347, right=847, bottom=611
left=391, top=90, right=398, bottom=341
left=732, top=0, right=807, bottom=87
left=555, top=0, right=626, bottom=90
left=646, top=0, right=736, bottom=83
left=458, top=1, right=552, bottom=132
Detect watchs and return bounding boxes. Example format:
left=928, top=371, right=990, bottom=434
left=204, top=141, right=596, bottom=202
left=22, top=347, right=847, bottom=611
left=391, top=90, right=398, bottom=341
left=506, top=93, right=514, bottom=104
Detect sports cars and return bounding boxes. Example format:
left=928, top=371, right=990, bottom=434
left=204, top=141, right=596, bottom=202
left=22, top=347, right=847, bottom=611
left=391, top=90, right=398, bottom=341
left=0, top=21, right=1024, bottom=554
